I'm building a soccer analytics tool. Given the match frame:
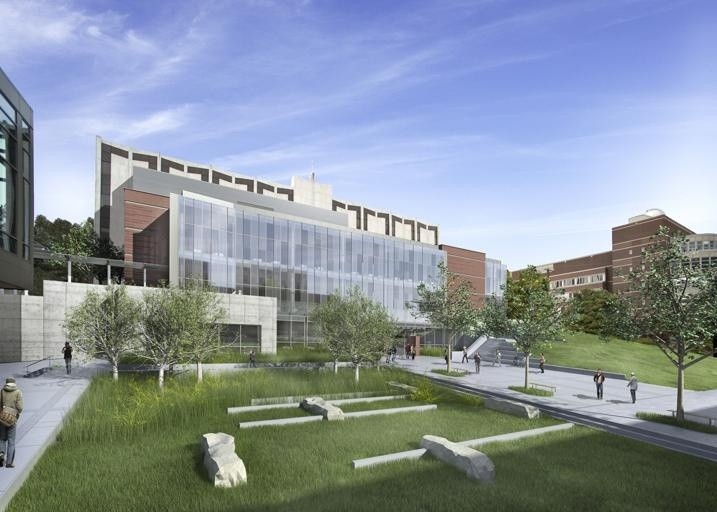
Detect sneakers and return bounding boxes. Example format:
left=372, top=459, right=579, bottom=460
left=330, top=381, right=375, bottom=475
left=0, top=453, right=4, bottom=467
left=6, top=463, right=16, bottom=468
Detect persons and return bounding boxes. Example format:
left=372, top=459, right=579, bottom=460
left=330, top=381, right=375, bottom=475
left=510, top=352, right=526, bottom=367
left=405, top=343, right=410, bottom=359
left=248, top=349, right=257, bottom=368
left=410, top=343, right=416, bottom=360
left=392, top=345, right=396, bottom=359
left=489, top=348, right=502, bottom=367
left=0, top=376, right=24, bottom=470
left=473, top=351, right=480, bottom=373
left=385, top=348, right=391, bottom=364
left=538, top=351, right=544, bottom=373
left=461, top=346, right=468, bottom=363
left=444, top=345, right=447, bottom=364
left=594, top=365, right=605, bottom=401
left=627, top=372, right=640, bottom=404
left=59, top=342, right=73, bottom=376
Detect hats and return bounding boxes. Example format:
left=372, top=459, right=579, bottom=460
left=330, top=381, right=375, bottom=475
left=5, top=378, right=16, bottom=383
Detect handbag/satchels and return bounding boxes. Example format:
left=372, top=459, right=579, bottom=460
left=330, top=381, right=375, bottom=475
left=0, top=406, right=18, bottom=428
